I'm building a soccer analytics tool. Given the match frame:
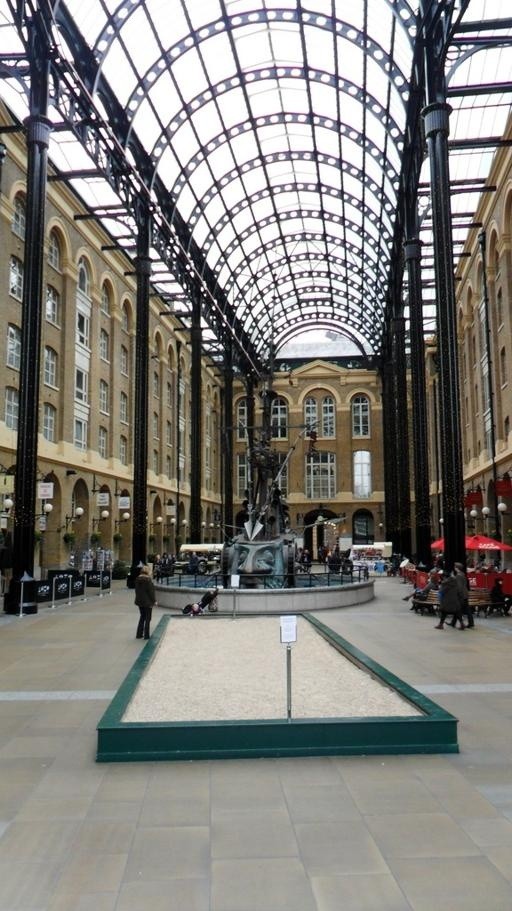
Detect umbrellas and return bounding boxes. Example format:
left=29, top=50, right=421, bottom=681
left=431, top=535, right=470, bottom=549
left=465, top=535, right=512, bottom=551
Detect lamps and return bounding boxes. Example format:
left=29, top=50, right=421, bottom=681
left=0, top=498, right=14, bottom=513
left=65, top=507, right=84, bottom=532
left=149, top=517, right=220, bottom=536
left=439, top=517, right=444, bottom=525
left=115, top=512, right=130, bottom=531
left=35, top=503, right=53, bottom=516
left=481, top=506, right=495, bottom=518
left=93, top=510, right=109, bottom=531
left=470, top=510, right=483, bottom=520
left=497, top=502, right=512, bottom=515
left=379, top=523, right=384, bottom=529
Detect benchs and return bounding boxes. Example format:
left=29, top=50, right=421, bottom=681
left=412, top=588, right=503, bottom=618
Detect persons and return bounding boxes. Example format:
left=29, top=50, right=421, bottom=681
left=133, top=566, right=158, bottom=640
left=383, top=553, right=512, bottom=631
left=297, top=545, right=328, bottom=573
left=153, top=551, right=199, bottom=579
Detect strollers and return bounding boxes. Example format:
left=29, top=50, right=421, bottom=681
left=182, top=589, right=220, bottom=616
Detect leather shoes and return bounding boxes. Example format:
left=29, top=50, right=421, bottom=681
left=435, top=621, right=474, bottom=629
